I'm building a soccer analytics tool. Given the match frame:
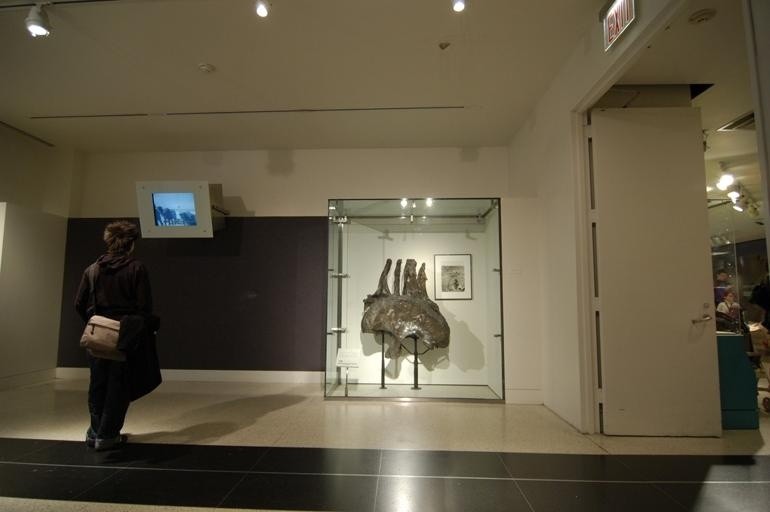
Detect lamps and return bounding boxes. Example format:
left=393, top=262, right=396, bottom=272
left=715, top=163, right=761, bottom=219
left=0, top=1, right=466, bottom=35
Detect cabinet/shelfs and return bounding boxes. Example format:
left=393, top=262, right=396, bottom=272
left=717, top=331, right=759, bottom=430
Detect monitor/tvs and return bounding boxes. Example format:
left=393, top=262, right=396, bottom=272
left=136, top=181, right=227, bottom=238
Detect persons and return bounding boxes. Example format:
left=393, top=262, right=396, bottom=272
left=714, top=268, right=734, bottom=305
left=750, top=274, right=770, bottom=350
left=74, top=222, right=165, bottom=450
left=717, top=288, right=753, bottom=352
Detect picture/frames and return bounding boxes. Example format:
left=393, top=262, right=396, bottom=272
left=434, top=253, right=472, bottom=299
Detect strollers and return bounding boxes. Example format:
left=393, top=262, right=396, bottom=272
left=716, top=310, right=770, bottom=413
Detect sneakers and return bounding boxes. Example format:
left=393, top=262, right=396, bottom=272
left=85, top=425, right=129, bottom=451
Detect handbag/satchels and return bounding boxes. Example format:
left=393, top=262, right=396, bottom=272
left=79, top=315, right=127, bottom=363
